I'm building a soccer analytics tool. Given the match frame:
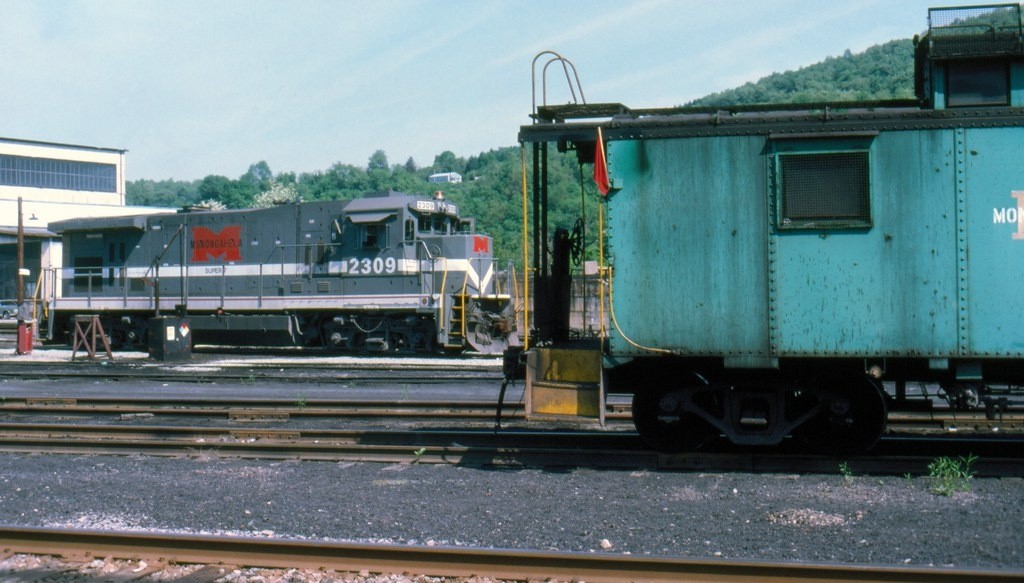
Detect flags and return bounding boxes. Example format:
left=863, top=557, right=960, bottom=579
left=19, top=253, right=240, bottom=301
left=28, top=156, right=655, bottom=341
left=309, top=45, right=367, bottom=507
left=594, top=132, right=610, bottom=196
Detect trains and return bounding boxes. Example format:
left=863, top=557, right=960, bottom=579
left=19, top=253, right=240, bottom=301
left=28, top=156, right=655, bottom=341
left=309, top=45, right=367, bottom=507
left=33, top=173, right=520, bottom=360
left=493, top=2, right=1024, bottom=461
